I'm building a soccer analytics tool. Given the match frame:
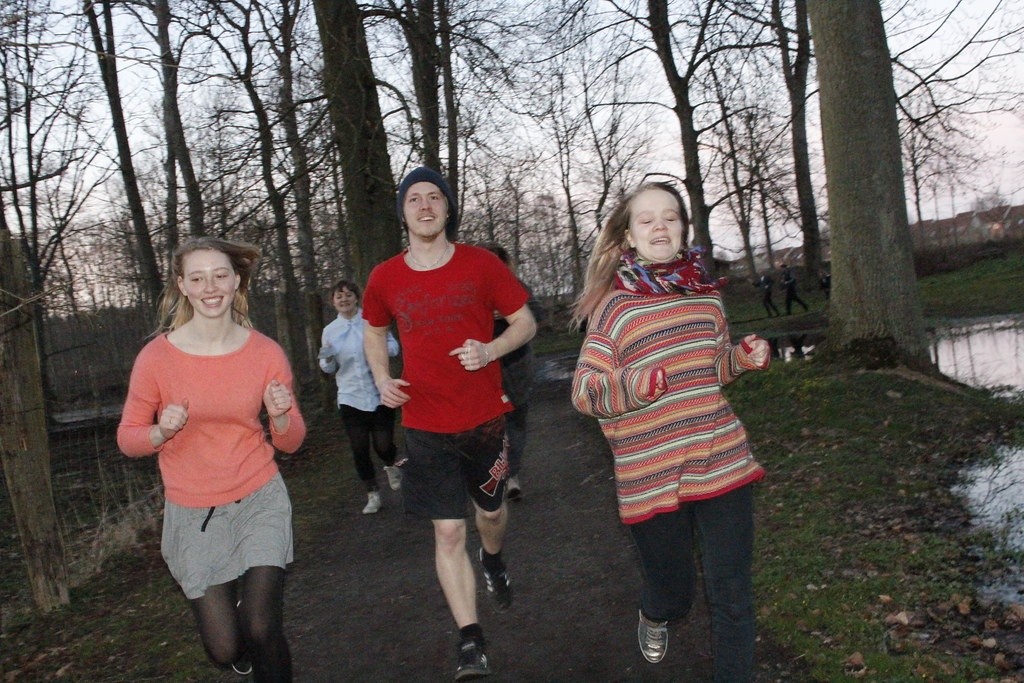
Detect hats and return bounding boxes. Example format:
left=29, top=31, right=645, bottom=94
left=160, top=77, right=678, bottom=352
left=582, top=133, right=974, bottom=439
left=396, top=167, right=456, bottom=224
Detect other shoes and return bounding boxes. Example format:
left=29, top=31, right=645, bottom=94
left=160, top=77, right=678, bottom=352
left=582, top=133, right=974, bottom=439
left=232, top=649, right=252, bottom=674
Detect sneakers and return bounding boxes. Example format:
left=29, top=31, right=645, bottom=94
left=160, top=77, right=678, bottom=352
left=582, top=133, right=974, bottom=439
left=637, top=609, right=669, bottom=663
left=383, top=464, right=401, bottom=490
left=455, top=629, right=492, bottom=681
left=362, top=491, right=382, bottom=514
left=476, top=547, right=513, bottom=614
left=506, top=474, right=522, bottom=501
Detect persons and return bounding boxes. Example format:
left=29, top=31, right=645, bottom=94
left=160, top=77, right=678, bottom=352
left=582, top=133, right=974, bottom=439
left=318, top=279, right=403, bottom=515
left=117, top=238, right=305, bottom=683
left=362, top=167, right=536, bottom=683
left=746, top=259, right=831, bottom=318
left=571, top=180, right=772, bottom=682
